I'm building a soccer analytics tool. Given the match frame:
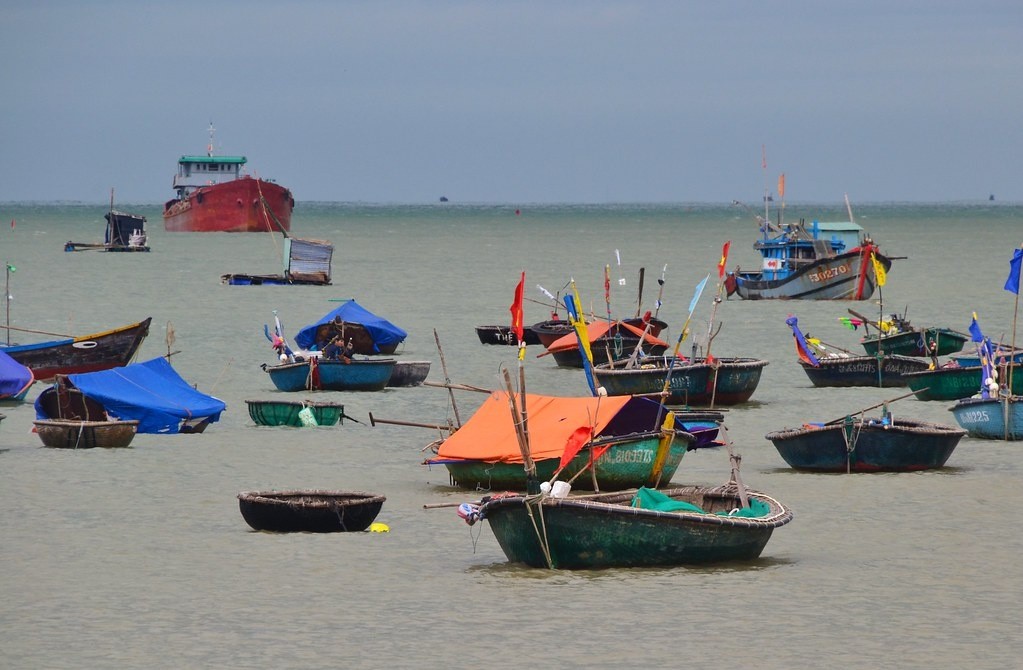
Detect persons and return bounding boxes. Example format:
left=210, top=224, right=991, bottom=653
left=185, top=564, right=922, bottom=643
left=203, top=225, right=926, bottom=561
left=326, top=336, right=353, bottom=360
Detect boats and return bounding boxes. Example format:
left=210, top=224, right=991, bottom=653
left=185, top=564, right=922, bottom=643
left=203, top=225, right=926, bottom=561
left=163, top=121, right=294, bottom=232
left=473, top=248, right=670, bottom=368
left=901, top=244, right=1023, bottom=440
left=563, top=240, right=771, bottom=404
left=723, top=143, right=907, bottom=300
left=838, top=308, right=969, bottom=357
left=245, top=399, right=364, bottom=427
left=459, top=271, right=795, bottom=570
left=765, top=387, right=969, bottom=474
left=785, top=249, right=931, bottom=388
left=64, top=189, right=151, bottom=252
left=257, top=299, right=431, bottom=391
left=33, top=419, right=139, bottom=449
left=368, top=329, right=733, bottom=490
left=220, top=170, right=334, bottom=286
left=236, top=490, right=386, bottom=532
left=34, top=322, right=228, bottom=435
left=0, top=261, right=153, bottom=402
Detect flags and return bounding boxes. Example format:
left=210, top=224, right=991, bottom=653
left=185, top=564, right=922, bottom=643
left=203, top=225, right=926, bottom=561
left=509, top=272, right=524, bottom=341
left=716, top=242, right=730, bottom=277
left=1004, top=248, right=1023, bottom=294
left=873, top=256, right=887, bottom=287
left=688, top=278, right=706, bottom=313
left=969, top=319, right=984, bottom=343
